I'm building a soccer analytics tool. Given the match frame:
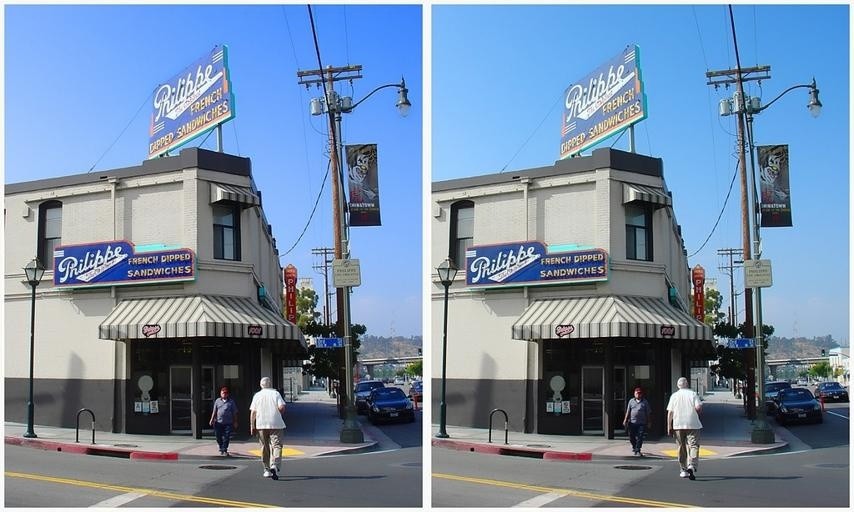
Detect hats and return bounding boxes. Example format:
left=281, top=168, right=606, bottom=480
left=221, top=387, right=228, bottom=394
left=634, top=388, right=642, bottom=394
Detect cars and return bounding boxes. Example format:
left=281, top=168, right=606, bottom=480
left=409, top=380, right=422, bottom=402
left=764, top=380, right=792, bottom=415
left=814, top=380, right=849, bottom=404
left=361, top=374, right=405, bottom=385
left=355, top=380, right=384, bottom=413
left=365, top=387, right=415, bottom=424
left=782, top=375, right=812, bottom=387
left=771, top=387, right=823, bottom=426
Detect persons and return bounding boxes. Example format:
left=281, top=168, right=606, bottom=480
left=622, top=386, right=652, bottom=456
left=247, top=376, right=287, bottom=480
left=208, top=385, right=240, bottom=456
left=665, top=376, right=704, bottom=480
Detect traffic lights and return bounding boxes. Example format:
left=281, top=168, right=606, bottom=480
left=821, top=350, right=825, bottom=356
left=417, top=347, right=421, bottom=357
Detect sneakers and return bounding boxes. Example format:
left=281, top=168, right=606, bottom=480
left=270, top=465, right=279, bottom=479
left=263, top=471, right=270, bottom=478
left=688, top=466, right=696, bottom=480
left=680, top=472, right=687, bottom=478
left=636, top=451, right=643, bottom=456
left=632, top=450, right=637, bottom=456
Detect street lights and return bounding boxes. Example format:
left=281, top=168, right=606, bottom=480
left=433, top=256, right=460, bottom=440
left=331, top=78, right=413, bottom=443
left=20, top=255, right=48, bottom=437
left=737, top=80, right=834, bottom=419
